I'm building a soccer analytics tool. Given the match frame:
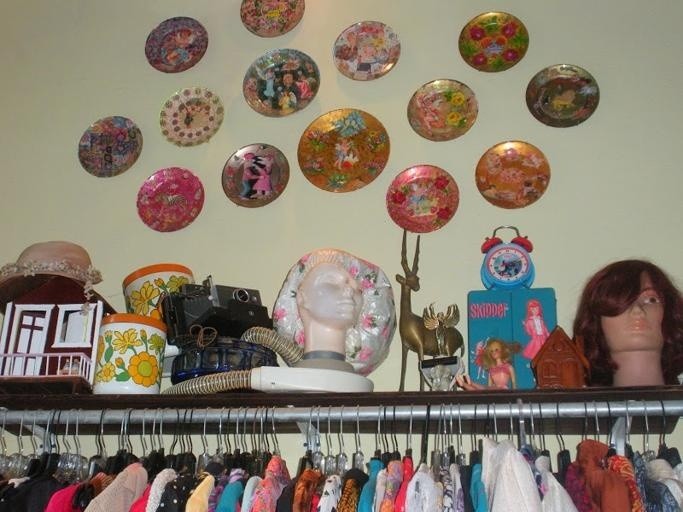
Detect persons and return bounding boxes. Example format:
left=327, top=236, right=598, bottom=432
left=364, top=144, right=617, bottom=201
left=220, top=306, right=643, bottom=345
left=297, top=262, right=363, bottom=361
left=279, top=91, right=291, bottom=109
left=423, top=304, right=459, bottom=357
left=572, top=261, right=683, bottom=389
left=262, top=68, right=275, bottom=97
left=520, top=300, right=550, bottom=360
left=456, top=338, right=521, bottom=392
left=184, top=105, right=196, bottom=128
left=241, top=155, right=273, bottom=200
left=296, top=80, right=314, bottom=99
left=333, top=138, right=352, bottom=169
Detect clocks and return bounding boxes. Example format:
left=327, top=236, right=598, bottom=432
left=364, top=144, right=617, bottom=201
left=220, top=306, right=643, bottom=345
left=480, top=225, right=537, bottom=289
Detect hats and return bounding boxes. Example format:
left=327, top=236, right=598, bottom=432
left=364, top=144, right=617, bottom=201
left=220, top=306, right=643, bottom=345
left=1, top=240, right=103, bottom=284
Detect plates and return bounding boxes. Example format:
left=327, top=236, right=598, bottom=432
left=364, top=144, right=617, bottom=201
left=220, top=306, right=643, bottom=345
left=78, top=116, right=144, bottom=179
left=386, top=167, right=461, bottom=234
left=145, top=16, right=210, bottom=72
left=526, top=65, right=599, bottom=129
left=136, top=167, right=205, bottom=232
left=239, top=1, right=306, bottom=38
left=459, top=12, right=529, bottom=71
left=474, top=142, right=549, bottom=209
left=296, top=110, right=390, bottom=193
left=159, top=87, right=225, bottom=148
left=243, top=49, right=322, bottom=119
left=222, top=143, right=290, bottom=209
left=407, top=79, right=478, bottom=142
left=329, top=21, right=401, bottom=80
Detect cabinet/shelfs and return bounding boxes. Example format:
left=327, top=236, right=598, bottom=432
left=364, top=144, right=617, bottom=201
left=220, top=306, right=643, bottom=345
left=468, top=289, right=557, bottom=389
